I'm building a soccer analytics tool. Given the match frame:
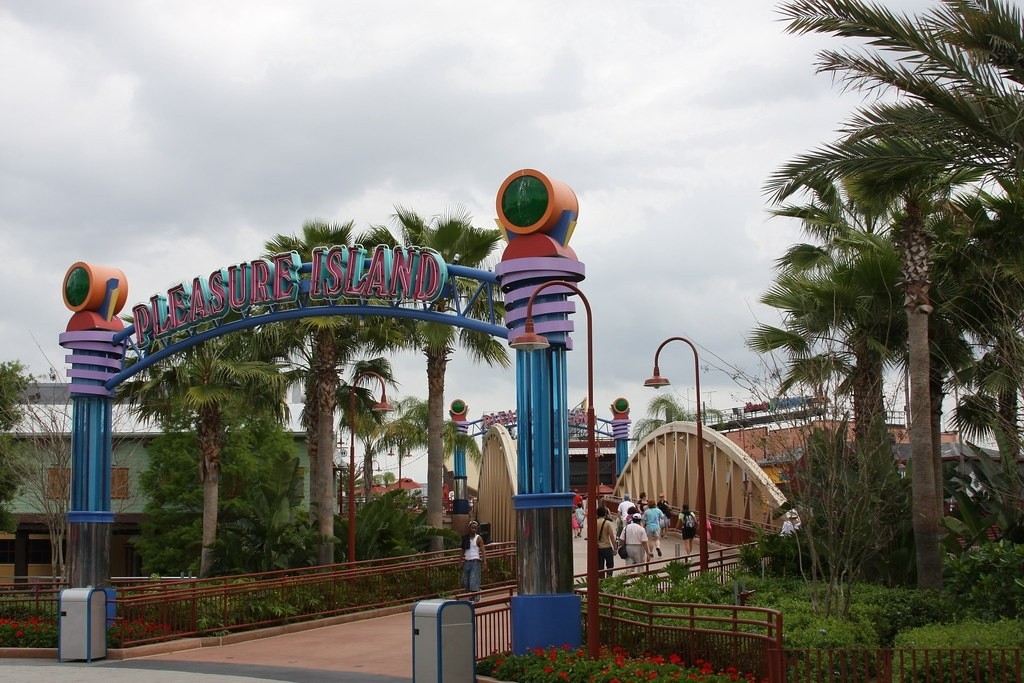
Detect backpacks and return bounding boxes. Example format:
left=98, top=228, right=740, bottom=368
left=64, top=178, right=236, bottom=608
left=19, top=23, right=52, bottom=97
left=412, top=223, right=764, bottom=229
left=681, top=512, right=697, bottom=529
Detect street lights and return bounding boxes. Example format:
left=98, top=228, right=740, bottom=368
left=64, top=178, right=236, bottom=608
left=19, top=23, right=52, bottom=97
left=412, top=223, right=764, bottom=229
left=643, top=337, right=710, bottom=577
left=348, top=372, right=395, bottom=587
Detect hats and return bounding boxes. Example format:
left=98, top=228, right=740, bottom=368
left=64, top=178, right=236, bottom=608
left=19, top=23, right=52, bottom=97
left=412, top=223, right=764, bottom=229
left=633, top=513, right=641, bottom=519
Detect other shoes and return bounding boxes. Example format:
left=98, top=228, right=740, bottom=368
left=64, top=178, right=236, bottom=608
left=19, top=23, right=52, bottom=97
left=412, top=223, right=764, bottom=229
left=656, top=548, right=662, bottom=556
left=650, top=553, right=654, bottom=558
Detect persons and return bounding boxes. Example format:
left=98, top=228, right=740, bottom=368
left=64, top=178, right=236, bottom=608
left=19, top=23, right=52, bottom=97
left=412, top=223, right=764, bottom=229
left=572, top=489, right=712, bottom=584
left=780, top=509, right=801, bottom=536
left=459, top=521, right=487, bottom=602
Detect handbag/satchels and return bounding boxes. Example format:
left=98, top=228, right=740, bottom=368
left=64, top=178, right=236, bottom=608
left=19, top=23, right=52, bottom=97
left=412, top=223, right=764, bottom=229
left=618, top=546, right=628, bottom=559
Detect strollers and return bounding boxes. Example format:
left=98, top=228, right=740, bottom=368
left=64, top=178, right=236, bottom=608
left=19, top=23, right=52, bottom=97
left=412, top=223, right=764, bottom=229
left=614, top=516, right=623, bottom=550
